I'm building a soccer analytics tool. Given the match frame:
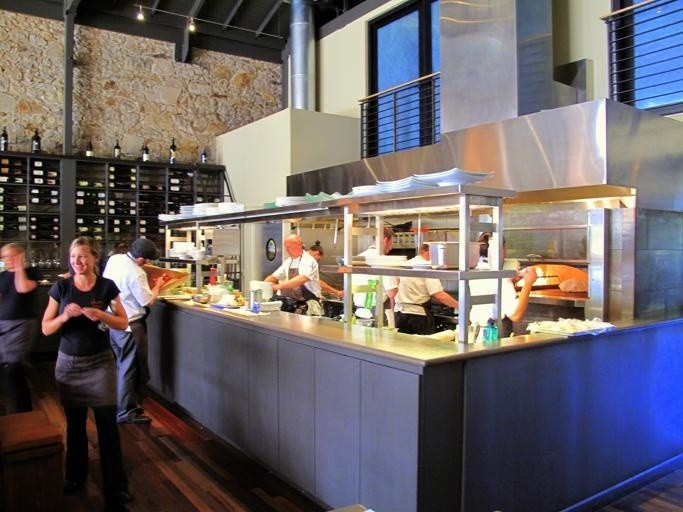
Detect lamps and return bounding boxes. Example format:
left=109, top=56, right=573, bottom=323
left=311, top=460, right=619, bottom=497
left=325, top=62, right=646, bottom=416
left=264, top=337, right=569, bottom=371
left=138, top=6, right=143, bottom=20
left=189, top=18, right=195, bottom=31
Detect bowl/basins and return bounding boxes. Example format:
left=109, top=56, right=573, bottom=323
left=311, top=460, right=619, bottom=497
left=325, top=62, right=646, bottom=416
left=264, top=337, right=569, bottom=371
left=193, top=294, right=209, bottom=303
left=425, top=242, right=484, bottom=269
left=175, top=247, right=205, bottom=260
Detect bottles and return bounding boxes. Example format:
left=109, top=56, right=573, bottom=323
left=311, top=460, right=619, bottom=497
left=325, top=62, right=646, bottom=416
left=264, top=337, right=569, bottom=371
left=483, top=318, right=498, bottom=341
left=0, top=126, right=208, bottom=166
left=209, top=268, right=216, bottom=300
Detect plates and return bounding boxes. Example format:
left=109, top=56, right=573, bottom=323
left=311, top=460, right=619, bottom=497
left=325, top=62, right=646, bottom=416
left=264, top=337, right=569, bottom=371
left=156, top=202, right=244, bottom=225
left=276, top=168, right=487, bottom=207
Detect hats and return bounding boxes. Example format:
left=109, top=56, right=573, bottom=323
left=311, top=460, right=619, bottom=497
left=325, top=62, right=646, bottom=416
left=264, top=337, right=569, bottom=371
left=132, top=239, right=161, bottom=260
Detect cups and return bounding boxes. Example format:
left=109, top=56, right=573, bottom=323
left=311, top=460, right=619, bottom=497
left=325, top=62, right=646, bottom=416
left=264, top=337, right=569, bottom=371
left=249, top=290, right=261, bottom=313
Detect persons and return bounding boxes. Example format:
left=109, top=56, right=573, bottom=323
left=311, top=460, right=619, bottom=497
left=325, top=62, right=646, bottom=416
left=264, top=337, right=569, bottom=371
left=38, top=233, right=134, bottom=504
left=466, top=229, right=538, bottom=342
left=0, top=239, right=42, bottom=415
left=349, top=223, right=402, bottom=333
left=390, top=242, right=458, bottom=337
left=96, top=237, right=171, bottom=426
left=305, top=238, right=344, bottom=303
left=261, top=233, right=326, bottom=317
left=99, top=241, right=128, bottom=279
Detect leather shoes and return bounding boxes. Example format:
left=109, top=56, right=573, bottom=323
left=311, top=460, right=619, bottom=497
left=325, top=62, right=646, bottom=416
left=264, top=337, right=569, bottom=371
left=128, top=413, right=153, bottom=425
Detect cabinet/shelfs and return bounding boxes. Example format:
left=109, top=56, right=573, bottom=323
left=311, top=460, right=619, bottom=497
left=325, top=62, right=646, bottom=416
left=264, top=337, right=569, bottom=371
left=0, top=152, right=237, bottom=287
